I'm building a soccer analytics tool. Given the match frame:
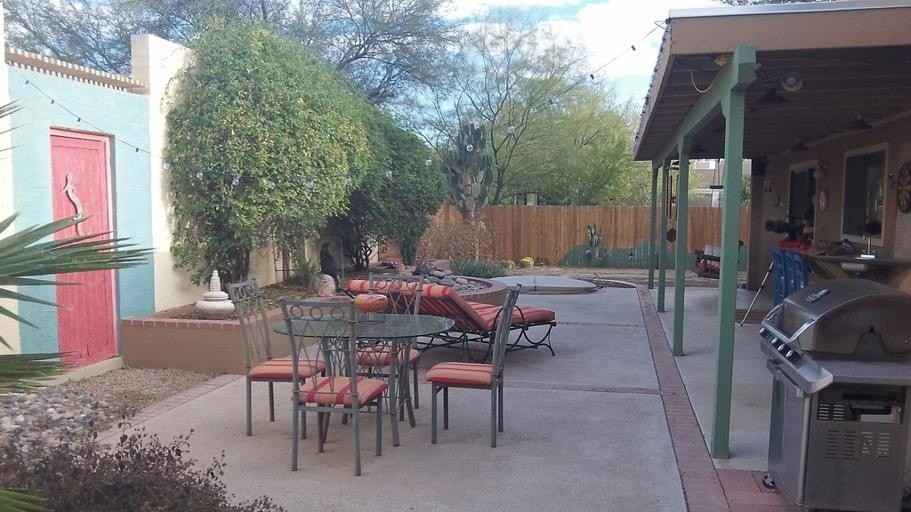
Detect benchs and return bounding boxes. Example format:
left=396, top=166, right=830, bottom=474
left=344, top=279, right=557, bottom=364
left=691, top=240, right=744, bottom=279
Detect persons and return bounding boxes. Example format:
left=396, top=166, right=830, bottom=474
left=802, top=194, right=816, bottom=243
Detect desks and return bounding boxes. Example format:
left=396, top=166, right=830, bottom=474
left=273, top=313, right=457, bottom=447
left=773, top=242, right=910, bottom=280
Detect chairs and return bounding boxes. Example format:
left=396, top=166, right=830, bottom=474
left=340, top=273, right=423, bottom=421
left=424, top=286, right=520, bottom=448
left=279, top=297, right=389, bottom=476
left=227, top=280, right=325, bottom=439
left=773, top=250, right=809, bottom=305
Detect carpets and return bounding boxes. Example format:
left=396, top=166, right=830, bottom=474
left=735, top=308, right=771, bottom=324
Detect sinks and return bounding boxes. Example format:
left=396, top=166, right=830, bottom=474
left=836, top=254, right=879, bottom=272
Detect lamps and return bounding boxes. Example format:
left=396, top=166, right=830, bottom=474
left=813, top=160, right=829, bottom=181
left=849, top=118, right=873, bottom=131
left=751, top=87, right=790, bottom=106
left=790, top=143, right=809, bottom=151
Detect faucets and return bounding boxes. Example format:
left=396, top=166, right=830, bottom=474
left=861, top=231, right=871, bottom=255
